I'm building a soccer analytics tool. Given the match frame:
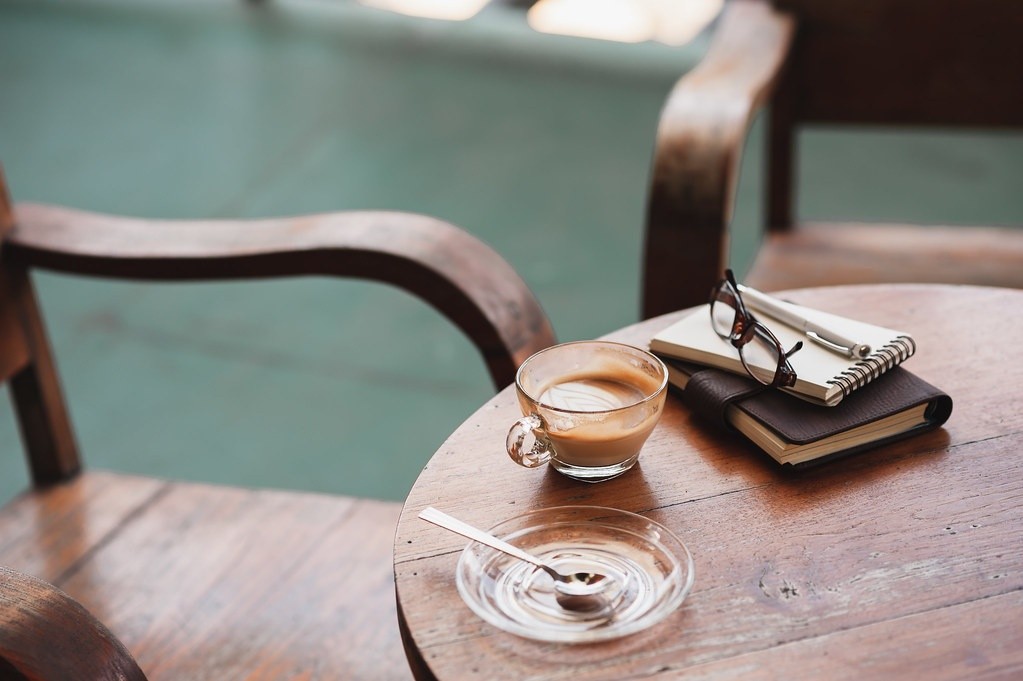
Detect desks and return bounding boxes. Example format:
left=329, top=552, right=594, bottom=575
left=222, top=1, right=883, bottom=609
left=392, top=283, right=1022, bottom=681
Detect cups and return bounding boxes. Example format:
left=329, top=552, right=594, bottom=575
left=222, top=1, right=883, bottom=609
left=507, top=340, right=668, bottom=483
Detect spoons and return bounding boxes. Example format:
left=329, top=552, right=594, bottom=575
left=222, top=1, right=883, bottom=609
left=418, top=506, right=617, bottom=597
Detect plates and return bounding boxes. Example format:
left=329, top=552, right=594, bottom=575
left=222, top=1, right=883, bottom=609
left=454, top=505, right=695, bottom=645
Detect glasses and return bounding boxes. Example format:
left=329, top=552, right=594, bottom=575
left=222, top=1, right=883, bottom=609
left=710, top=268, right=803, bottom=387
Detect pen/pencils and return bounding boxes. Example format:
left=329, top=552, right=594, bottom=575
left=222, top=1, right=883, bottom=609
left=726, top=278, right=871, bottom=360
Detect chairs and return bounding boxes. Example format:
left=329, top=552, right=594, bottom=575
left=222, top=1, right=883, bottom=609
left=1, top=159, right=558, bottom=681
left=640, top=0, right=1023, bottom=317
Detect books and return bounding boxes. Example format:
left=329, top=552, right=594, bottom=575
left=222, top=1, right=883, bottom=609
left=642, top=354, right=952, bottom=472
left=648, top=290, right=915, bottom=407
